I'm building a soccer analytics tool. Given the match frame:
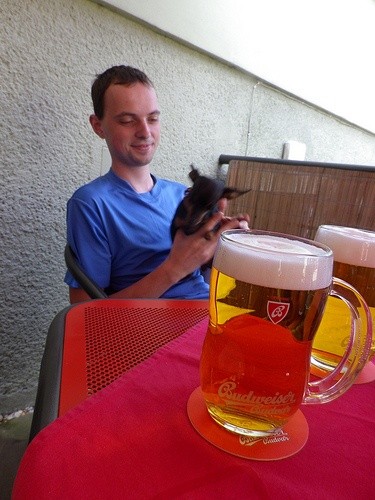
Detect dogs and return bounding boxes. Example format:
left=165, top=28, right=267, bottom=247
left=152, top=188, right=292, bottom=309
left=169, top=165, right=254, bottom=280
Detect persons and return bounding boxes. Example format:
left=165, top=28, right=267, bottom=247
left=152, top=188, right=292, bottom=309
left=62, top=65, right=252, bottom=301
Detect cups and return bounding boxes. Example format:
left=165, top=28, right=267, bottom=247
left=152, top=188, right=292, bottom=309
left=308, top=224, right=375, bottom=375
left=200, top=229, right=374, bottom=441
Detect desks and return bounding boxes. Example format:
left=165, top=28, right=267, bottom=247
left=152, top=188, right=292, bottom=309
left=11, top=296, right=374, bottom=500
